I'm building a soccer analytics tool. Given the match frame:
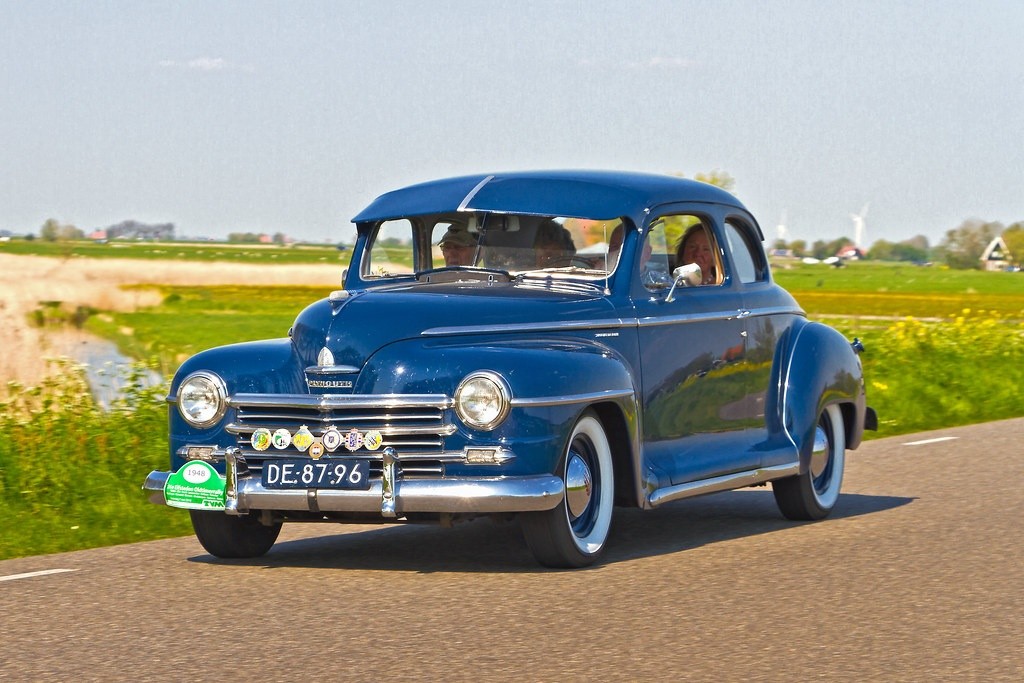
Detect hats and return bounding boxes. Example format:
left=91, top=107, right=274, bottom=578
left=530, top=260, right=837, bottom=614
left=437, top=225, right=479, bottom=244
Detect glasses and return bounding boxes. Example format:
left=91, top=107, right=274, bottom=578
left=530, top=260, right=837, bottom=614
left=439, top=242, right=465, bottom=251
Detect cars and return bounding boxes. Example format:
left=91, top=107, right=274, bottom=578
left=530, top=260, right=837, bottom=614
left=142, top=169, right=878, bottom=570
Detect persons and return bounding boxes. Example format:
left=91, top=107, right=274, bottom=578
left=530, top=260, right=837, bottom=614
left=595, top=223, right=676, bottom=289
left=532, top=221, right=590, bottom=271
left=437, top=225, right=485, bottom=269
left=668, top=223, right=720, bottom=285
